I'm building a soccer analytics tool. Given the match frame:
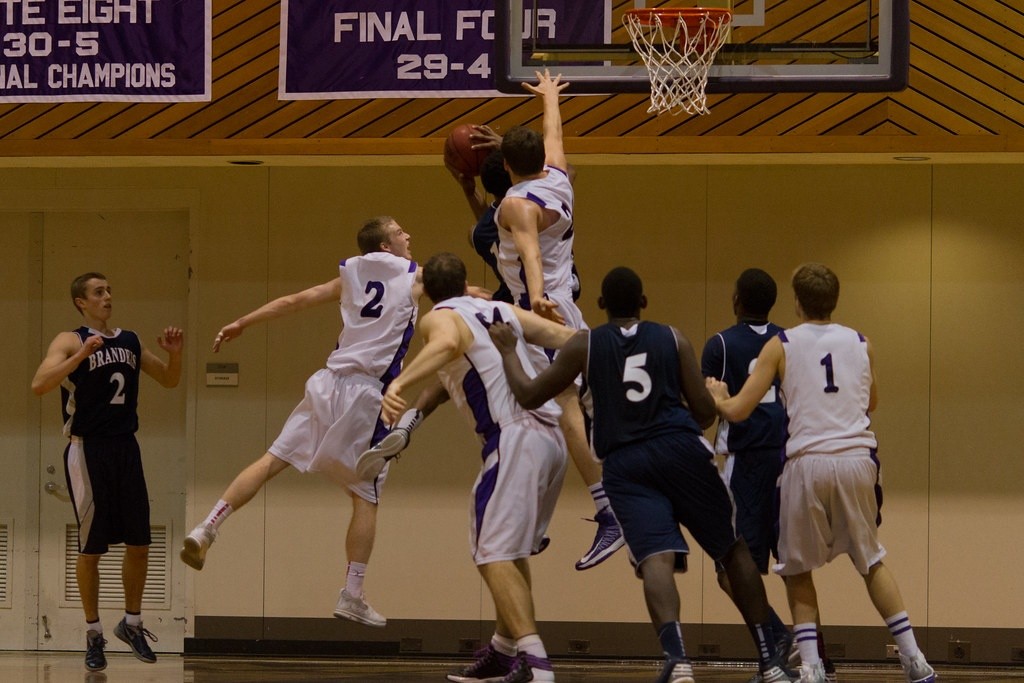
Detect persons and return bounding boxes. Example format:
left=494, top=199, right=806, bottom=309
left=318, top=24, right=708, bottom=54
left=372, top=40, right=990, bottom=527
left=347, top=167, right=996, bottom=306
left=31, top=272, right=184, bottom=671
left=178, top=66, right=938, bottom=683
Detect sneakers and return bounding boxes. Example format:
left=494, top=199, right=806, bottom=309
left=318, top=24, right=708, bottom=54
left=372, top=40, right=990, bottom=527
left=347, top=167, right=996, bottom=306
left=657, top=651, right=694, bottom=683
left=85, top=630, right=108, bottom=671
left=575, top=514, right=627, bottom=571
left=446, top=642, right=517, bottom=683
left=760, top=622, right=797, bottom=683
left=333, top=587, right=386, bottom=626
left=898, top=647, right=936, bottom=683
left=793, top=658, right=824, bottom=683
left=532, top=535, right=550, bottom=554
left=180, top=522, right=217, bottom=570
left=113, top=616, right=158, bottom=663
left=479, top=651, right=556, bottom=683
left=827, top=669, right=837, bottom=681
left=355, top=426, right=413, bottom=481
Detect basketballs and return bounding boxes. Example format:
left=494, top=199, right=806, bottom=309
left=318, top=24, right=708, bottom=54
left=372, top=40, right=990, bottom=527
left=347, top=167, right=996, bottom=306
left=444, top=123, right=491, bottom=176
